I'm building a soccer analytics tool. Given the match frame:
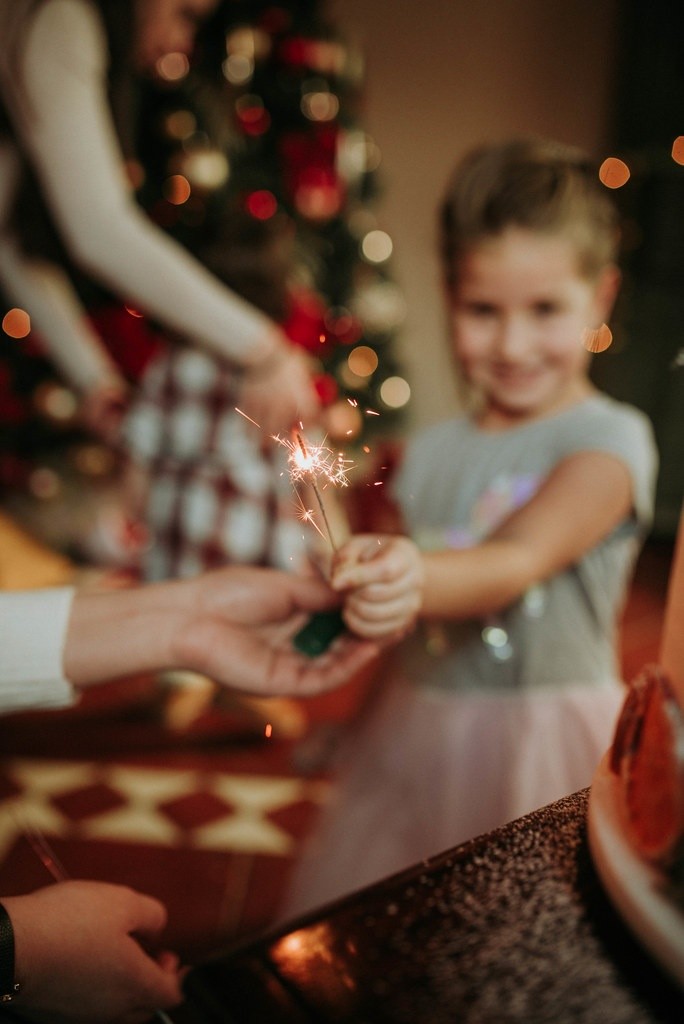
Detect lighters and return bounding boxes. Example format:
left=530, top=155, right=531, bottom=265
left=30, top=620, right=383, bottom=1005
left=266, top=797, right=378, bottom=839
left=293, top=612, right=345, bottom=657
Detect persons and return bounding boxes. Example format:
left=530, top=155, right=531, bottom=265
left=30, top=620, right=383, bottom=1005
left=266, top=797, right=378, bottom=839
left=0, top=0, right=684, bottom=1024
